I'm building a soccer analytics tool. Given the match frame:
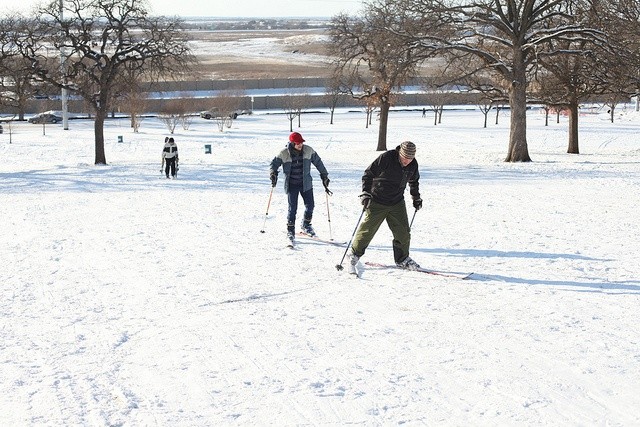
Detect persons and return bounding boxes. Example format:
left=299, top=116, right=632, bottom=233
left=163, top=138, right=178, bottom=179
left=269, top=133, right=330, bottom=249
left=159, top=137, right=179, bottom=173
left=347, top=141, right=423, bottom=274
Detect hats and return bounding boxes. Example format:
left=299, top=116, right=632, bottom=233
left=399, top=141, right=416, bottom=159
left=289, top=132, right=305, bottom=144
left=168, top=138, right=174, bottom=143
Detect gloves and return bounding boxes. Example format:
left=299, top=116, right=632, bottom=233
left=412, top=194, right=422, bottom=210
left=358, top=191, right=373, bottom=209
left=322, top=176, right=330, bottom=187
left=270, top=173, right=278, bottom=187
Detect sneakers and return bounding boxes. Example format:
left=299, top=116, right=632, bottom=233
left=287, top=223, right=295, bottom=239
left=303, top=219, right=315, bottom=236
left=350, top=253, right=358, bottom=265
left=395, top=256, right=420, bottom=270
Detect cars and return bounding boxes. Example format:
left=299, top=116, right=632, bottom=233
left=199, top=106, right=242, bottom=119
left=28, top=113, right=63, bottom=125
